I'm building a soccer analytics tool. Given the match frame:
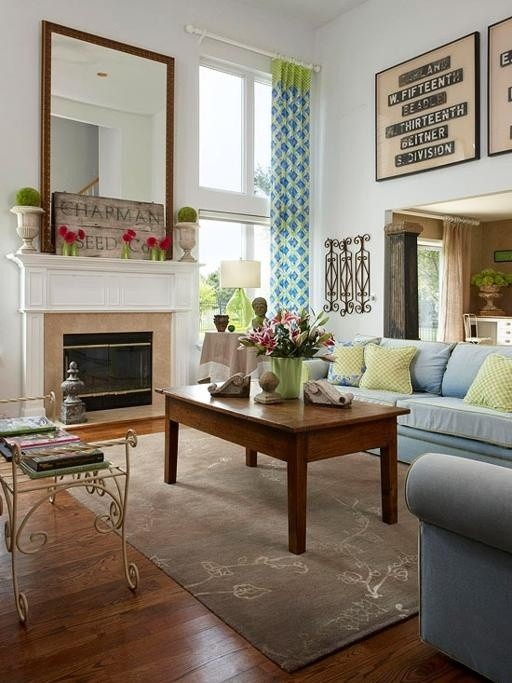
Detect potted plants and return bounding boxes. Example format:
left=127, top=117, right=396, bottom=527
left=469, top=265, right=512, bottom=315
left=7, top=185, right=47, bottom=253
left=173, top=205, right=202, bottom=263
left=213, top=290, right=230, bottom=332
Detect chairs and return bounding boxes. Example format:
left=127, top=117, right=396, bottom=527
left=461, top=312, right=492, bottom=345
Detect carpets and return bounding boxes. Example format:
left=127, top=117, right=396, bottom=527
left=50, top=427, right=421, bottom=676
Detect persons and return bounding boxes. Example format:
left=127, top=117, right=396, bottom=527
left=250, top=297, right=271, bottom=330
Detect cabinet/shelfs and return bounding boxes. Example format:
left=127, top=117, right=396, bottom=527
left=0, top=390, right=142, bottom=626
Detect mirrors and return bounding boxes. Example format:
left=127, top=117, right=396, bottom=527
left=38, top=18, right=176, bottom=261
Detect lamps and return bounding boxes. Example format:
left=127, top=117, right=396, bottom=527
left=219, top=256, right=262, bottom=332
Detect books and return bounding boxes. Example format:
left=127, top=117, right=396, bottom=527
left=1, top=413, right=110, bottom=480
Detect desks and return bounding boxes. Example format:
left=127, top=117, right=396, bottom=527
left=466, top=315, right=512, bottom=345
left=196, top=330, right=272, bottom=383
left=153, top=380, right=412, bottom=556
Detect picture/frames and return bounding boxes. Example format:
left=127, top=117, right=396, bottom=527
left=493, top=249, right=512, bottom=262
left=487, top=16, right=512, bottom=160
left=372, top=30, right=482, bottom=184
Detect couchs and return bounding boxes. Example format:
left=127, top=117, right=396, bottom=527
left=399, top=450, right=512, bottom=683
left=303, top=337, right=512, bottom=468
left=326, top=345, right=382, bottom=388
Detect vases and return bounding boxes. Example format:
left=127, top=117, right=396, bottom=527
left=123, top=244, right=129, bottom=259
left=151, top=248, right=158, bottom=260
left=71, top=242, right=79, bottom=256
left=62, top=238, right=69, bottom=255
left=159, top=249, right=167, bottom=261
left=272, top=356, right=303, bottom=400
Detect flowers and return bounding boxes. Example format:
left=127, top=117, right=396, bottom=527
left=236, top=305, right=340, bottom=363
left=57, top=223, right=86, bottom=244
left=146, top=235, right=170, bottom=251
left=120, top=228, right=137, bottom=246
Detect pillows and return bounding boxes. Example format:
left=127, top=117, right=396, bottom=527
left=359, top=342, right=420, bottom=396
left=439, top=343, right=512, bottom=400
left=380, top=335, right=458, bottom=397
left=462, top=352, right=512, bottom=414
